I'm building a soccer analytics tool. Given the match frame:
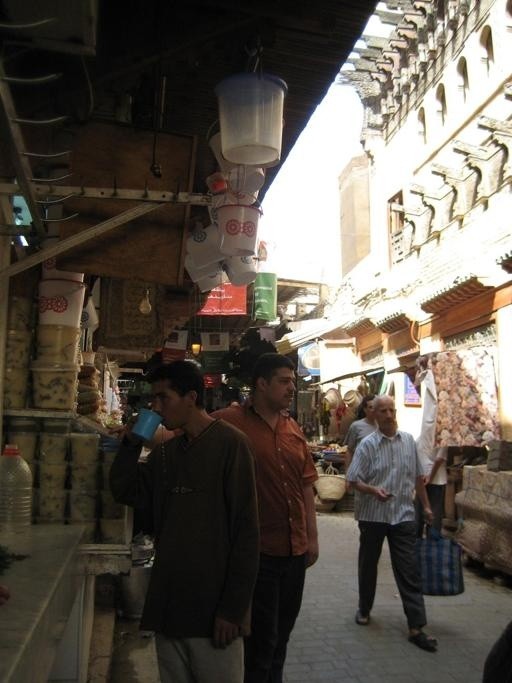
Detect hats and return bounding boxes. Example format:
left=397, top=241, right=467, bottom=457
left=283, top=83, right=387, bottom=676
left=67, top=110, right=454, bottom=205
left=323, top=387, right=363, bottom=409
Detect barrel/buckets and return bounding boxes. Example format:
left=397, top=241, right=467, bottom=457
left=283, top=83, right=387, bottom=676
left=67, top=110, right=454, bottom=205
left=120, top=558, right=157, bottom=620
left=4, top=276, right=127, bottom=546
left=181, top=73, right=288, bottom=294
left=314, top=465, right=349, bottom=502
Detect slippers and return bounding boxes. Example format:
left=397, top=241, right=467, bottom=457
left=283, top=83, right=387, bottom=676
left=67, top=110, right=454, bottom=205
left=410, top=631, right=439, bottom=648
left=355, top=608, right=369, bottom=625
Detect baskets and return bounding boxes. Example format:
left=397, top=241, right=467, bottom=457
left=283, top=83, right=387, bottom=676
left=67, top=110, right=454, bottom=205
left=315, top=474, right=347, bottom=501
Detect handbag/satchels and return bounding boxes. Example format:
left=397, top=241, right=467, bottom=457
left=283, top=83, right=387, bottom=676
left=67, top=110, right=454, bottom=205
left=417, top=527, right=464, bottom=596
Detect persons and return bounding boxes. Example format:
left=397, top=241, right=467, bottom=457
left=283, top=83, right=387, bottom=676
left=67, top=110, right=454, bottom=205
left=109, top=353, right=319, bottom=683
left=415, top=425, right=448, bottom=541
left=344, top=394, right=380, bottom=519
left=347, top=395, right=439, bottom=651
left=108, top=360, right=260, bottom=683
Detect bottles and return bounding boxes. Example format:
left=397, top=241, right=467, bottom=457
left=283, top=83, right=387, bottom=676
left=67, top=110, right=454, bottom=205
left=0, top=444, right=33, bottom=559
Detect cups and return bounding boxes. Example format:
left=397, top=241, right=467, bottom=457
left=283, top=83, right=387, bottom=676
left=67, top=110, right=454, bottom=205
left=132, top=408, right=164, bottom=440
